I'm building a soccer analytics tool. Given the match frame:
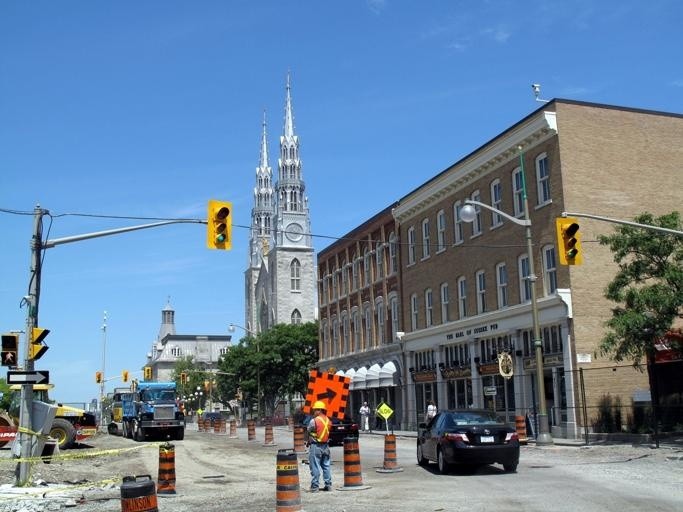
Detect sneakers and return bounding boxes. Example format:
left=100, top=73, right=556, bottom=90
left=319, top=486, right=331, bottom=491
left=303, top=488, right=319, bottom=493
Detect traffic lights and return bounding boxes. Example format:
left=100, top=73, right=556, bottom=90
left=121, top=370, right=128, bottom=383
left=556, top=215, right=582, bottom=265
left=144, top=367, right=152, bottom=380
left=95, top=371, right=102, bottom=383
left=28, top=325, right=51, bottom=360
left=207, top=200, right=232, bottom=251
left=0, top=333, right=18, bottom=369
left=180, top=373, right=186, bottom=385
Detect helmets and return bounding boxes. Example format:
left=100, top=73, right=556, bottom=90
left=311, top=401, right=326, bottom=410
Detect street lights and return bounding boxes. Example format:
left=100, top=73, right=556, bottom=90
left=456, top=198, right=556, bottom=449
left=96, top=309, right=109, bottom=427
left=225, top=320, right=263, bottom=418
left=176, top=385, right=204, bottom=416
left=104, top=403, right=114, bottom=425
left=188, top=357, right=213, bottom=413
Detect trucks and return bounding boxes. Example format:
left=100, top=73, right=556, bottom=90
left=117, top=379, right=185, bottom=442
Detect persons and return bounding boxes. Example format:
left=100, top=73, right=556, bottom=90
left=426, top=399, right=437, bottom=421
left=306, top=401, right=334, bottom=493
left=358, top=401, right=370, bottom=432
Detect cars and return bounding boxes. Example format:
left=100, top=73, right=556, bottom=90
left=296, top=410, right=361, bottom=448
left=413, top=406, right=523, bottom=477
left=199, top=410, right=225, bottom=426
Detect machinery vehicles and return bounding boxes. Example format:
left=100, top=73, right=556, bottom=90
left=0, top=382, right=99, bottom=452
left=107, top=386, right=131, bottom=436
